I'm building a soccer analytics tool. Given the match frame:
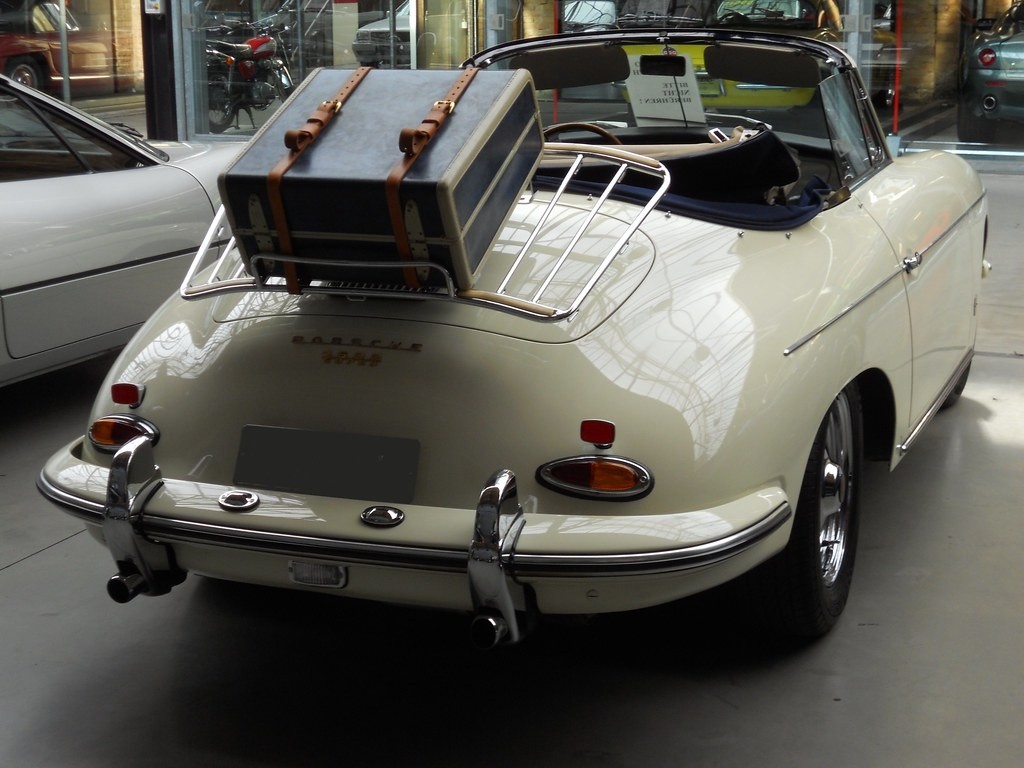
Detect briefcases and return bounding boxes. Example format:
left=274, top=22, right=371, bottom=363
left=218, top=66, right=545, bottom=291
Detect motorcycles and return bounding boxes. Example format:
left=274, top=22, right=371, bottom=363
left=206, top=5, right=296, bottom=134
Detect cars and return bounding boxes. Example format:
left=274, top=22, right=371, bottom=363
left=34, top=13, right=992, bottom=659
left=351, top=0, right=411, bottom=67
left=1, top=75, right=257, bottom=391
left=0, top=0, right=111, bottom=103
left=954, top=2, right=1024, bottom=135
